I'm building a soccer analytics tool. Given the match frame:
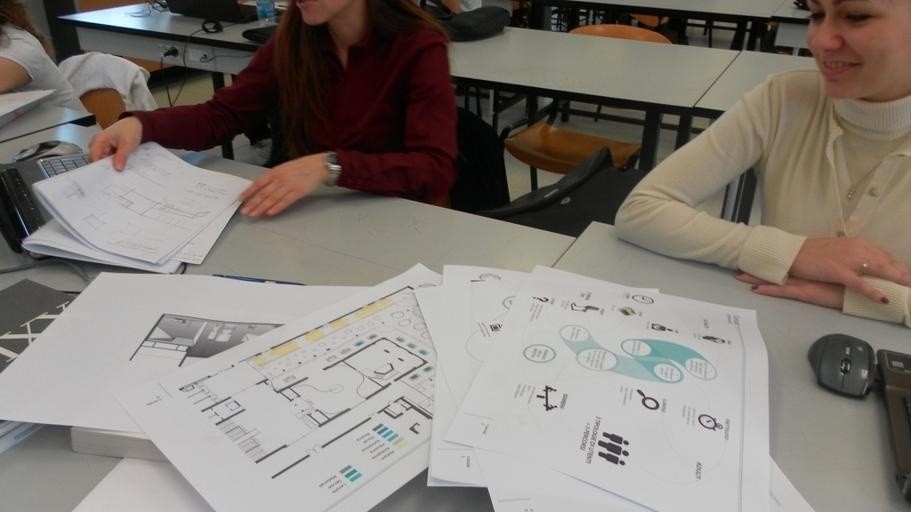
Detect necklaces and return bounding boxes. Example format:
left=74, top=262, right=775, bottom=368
left=843, top=156, right=882, bottom=199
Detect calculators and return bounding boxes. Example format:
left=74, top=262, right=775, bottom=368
left=1, top=152, right=91, bottom=260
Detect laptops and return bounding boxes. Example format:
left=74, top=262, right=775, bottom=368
left=0, top=153, right=107, bottom=261
left=877, top=349, right=911, bottom=503
left=166, top=0, right=286, bottom=24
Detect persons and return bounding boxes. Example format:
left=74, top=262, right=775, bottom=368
left=2, top=1, right=89, bottom=115
left=443, top=1, right=514, bottom=17
left=90, top=1, right=458, bottom=221
left=616, top=1, right=911, bottom=329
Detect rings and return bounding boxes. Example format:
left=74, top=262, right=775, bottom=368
left=862, top=260, right=870, bottom=274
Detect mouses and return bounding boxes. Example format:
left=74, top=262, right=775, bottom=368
left=808, top=334, right=875, bottom=399
left=13, top=141, right=83, bottom=164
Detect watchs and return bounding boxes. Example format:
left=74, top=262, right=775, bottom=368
left=323, top=151, right=343, bottom=186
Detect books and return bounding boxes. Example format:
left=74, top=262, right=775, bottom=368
left=70, top=274, right=307, bottom=462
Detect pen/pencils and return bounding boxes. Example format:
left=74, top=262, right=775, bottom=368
left=210, top=272, right=303, bottom=286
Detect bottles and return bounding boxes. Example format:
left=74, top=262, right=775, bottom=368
left=256, top=0, right=276, bottom=26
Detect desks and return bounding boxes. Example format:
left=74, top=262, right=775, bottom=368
left=404, top=219, right=909, bottom=509
left=0, top=123, right=573, bottom=511
left=59, top=4, right=739, bottom=200
left=772, top=2, right=815, bottom=56
left=699, top=51, right=827, bottom=230
left=0, top=89, right=97, bottom=144
left=602, top=2, right=788, bottom=55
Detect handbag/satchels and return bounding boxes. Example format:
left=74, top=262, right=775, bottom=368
left=418, top=0, right=510, bottom=43
